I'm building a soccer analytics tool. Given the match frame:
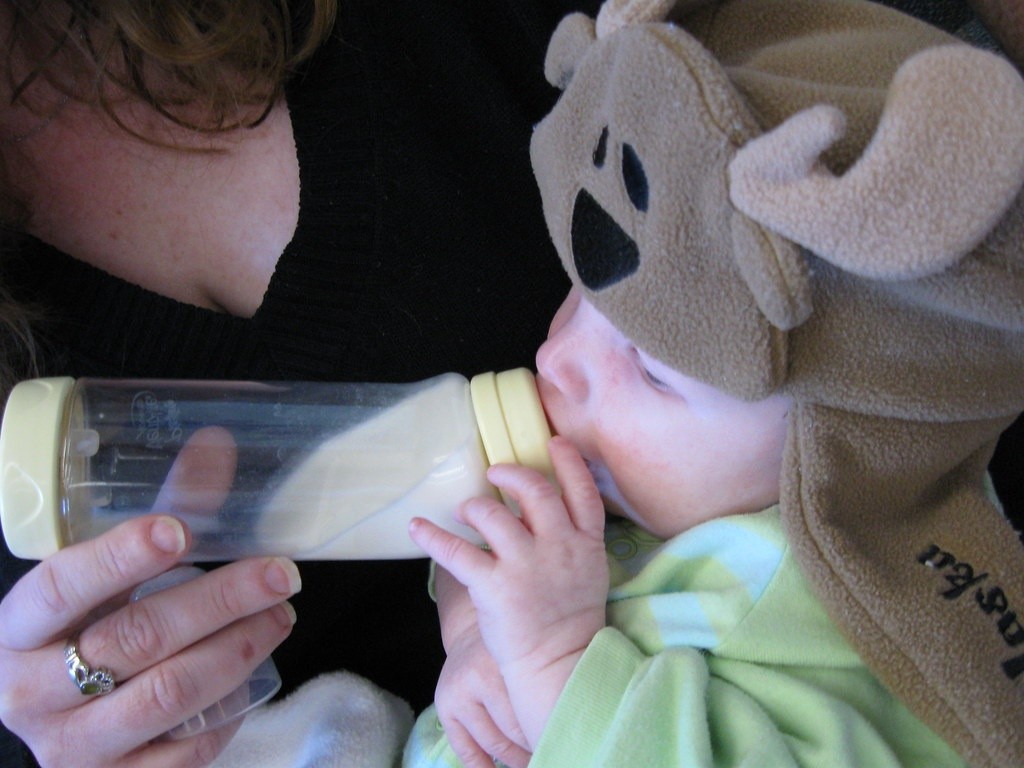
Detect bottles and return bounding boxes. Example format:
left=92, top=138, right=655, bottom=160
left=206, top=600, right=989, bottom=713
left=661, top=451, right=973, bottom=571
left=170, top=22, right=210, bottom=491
left=0, top=367, right=561, bottom=559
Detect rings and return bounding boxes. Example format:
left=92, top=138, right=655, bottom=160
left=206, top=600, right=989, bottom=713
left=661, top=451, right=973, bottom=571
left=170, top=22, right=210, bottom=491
left=63, top=630, right=117, bottom=695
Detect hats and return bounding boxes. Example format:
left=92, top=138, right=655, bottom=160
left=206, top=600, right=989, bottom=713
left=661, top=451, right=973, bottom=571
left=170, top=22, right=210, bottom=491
left=529, top=0, right=1024, bottom=768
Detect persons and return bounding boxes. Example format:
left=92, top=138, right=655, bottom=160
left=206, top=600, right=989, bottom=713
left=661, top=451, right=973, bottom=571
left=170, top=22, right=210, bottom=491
left=185, top=1, right=1024, bottom=768
left=1, top=1, right=1024, bottom=768
